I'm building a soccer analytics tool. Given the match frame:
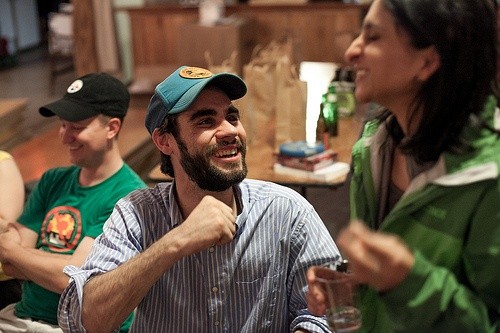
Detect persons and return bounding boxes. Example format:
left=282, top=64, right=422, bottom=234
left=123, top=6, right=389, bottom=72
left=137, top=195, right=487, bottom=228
left=0, top=72, right=149, bottom=333
left=306, top=0, right=500, bottom=333
left=57, top=65, right=344, bottom=333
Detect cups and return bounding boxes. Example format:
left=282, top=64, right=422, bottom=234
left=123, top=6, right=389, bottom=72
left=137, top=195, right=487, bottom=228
left=314, top=261, right=363, bottom=333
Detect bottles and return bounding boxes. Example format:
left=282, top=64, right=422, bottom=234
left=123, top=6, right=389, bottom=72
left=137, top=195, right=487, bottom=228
left=317, top=86, right=338, bottom=139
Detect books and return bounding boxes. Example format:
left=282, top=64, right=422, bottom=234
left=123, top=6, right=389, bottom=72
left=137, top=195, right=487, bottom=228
left=271, top=138, right=350, bottom=187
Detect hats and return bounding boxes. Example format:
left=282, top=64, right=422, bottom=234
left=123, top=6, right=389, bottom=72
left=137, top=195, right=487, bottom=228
left=38, top=71, right=130, bottom=122
left=145, top=64, right=247, bottom=137
